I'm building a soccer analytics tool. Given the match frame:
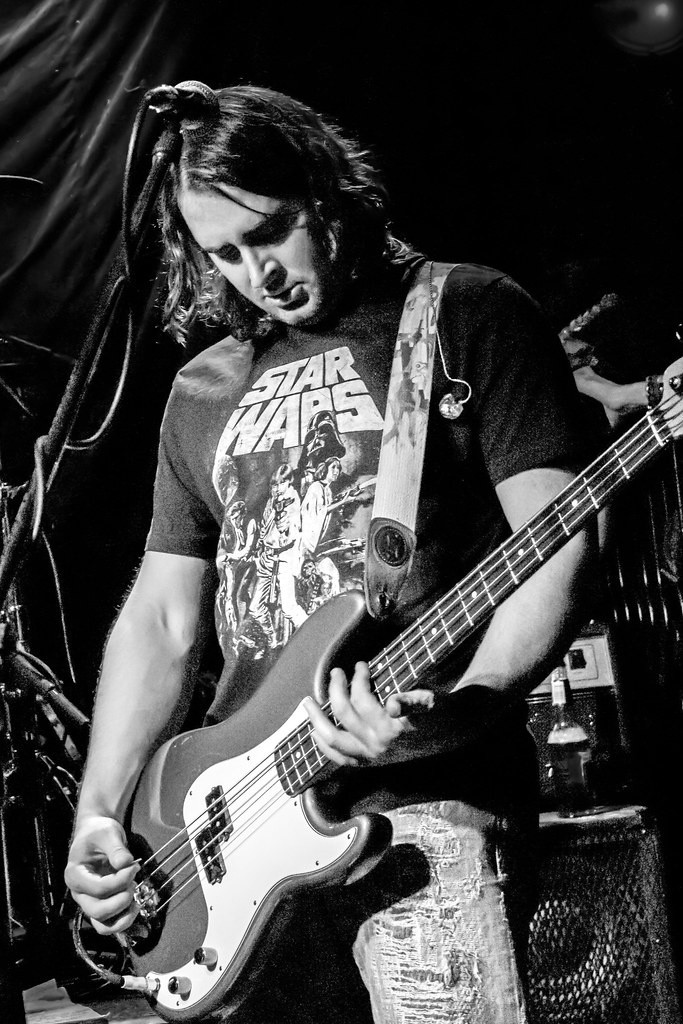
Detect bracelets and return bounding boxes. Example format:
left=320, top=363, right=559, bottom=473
left=645, top=375, right=661, bottom=406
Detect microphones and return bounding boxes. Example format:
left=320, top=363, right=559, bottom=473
left=145, top=80, right=220, bottom=140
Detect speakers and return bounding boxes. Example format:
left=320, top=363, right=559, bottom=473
left=502, top=804, right=683, bottom=1024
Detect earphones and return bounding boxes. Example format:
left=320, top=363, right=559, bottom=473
left=439, top=394, right=463, bottom=420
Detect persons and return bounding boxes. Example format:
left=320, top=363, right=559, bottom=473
left=61, top=84, right=602, bottom=1022
left=565, top=362, right=663, bottom=414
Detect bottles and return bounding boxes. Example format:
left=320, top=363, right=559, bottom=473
left=545, top=658, right=602, bottom=819
